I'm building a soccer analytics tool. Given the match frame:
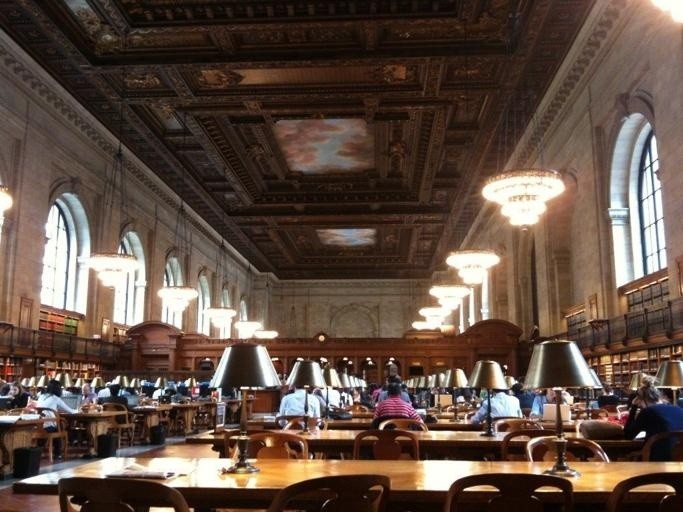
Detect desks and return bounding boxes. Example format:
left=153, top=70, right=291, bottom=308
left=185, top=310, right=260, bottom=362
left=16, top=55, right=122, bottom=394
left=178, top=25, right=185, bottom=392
left=1, top=395, right=255, bottom=475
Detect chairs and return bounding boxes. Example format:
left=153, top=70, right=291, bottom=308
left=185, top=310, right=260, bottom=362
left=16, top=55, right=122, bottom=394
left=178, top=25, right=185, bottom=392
left=57, top=404, right=682, bottom=511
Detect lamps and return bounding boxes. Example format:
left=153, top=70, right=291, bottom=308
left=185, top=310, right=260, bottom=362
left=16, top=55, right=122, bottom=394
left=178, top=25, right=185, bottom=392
left=156, top=17, right=199, bottom=314
left=87, top=28, right=140, bottom=291
left=411, top=152, right=471, bottom=331
left=478, top=13, right=577, bottom=233
left=20, top=372, right=198, bottom=405
left=0, top=151, right=12, bottom=230
left=630, top=372, right=646, bottom=391
left=444, top=57, right=501, bottom=286
left=287, top=359, right=326, bottom=437
left=323, top=366, right=368, bottom=423
left=205, top=110, right=278, bottom=341
left=208, top=343, right=282, bottom=476
left=520, top=339, right=604, bottom=477
left=653, top=359, right=683, bottom=406
left=402, top=366, right=467, bottom=422
left=466, top=360, right=510, bottom=437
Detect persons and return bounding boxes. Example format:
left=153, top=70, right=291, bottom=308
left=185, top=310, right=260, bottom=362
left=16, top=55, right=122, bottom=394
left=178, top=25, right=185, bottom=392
left=624, top=380, right=683, bottom=461
left=0, top=376, right=220, bottom=463
left=627, top=377, right=655, bottom=412
left=597, top=384, right=621, bottom=412
left=274, top=378, right=574, bottom=433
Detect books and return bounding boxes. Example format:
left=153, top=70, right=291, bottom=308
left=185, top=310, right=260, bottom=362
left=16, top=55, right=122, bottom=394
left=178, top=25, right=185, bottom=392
left=586, top=343, right=683, bottom=385
left=0, top=356, right=100, bottom=382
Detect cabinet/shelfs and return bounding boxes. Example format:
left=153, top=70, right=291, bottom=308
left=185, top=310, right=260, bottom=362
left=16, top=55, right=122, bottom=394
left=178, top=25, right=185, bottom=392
left=578, top=330, right=683, bottom=397
